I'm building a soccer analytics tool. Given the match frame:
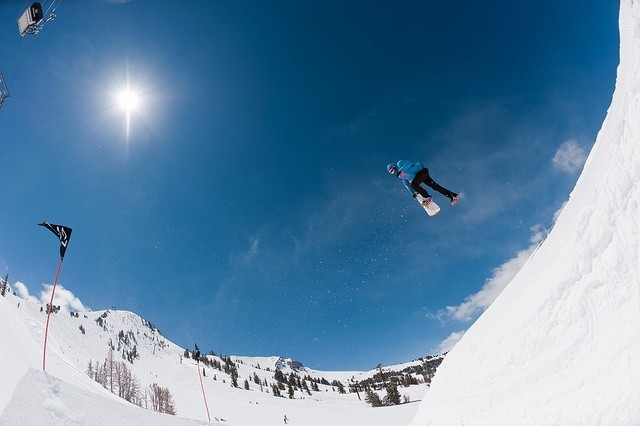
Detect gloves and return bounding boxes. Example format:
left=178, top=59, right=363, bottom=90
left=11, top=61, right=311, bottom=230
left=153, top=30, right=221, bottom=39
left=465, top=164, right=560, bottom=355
left=412, top=193, right=418, bottom=198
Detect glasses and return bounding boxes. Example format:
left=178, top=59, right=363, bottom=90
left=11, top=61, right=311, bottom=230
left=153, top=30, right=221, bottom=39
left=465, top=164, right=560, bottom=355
left=389, top=168, right=395, bottom=174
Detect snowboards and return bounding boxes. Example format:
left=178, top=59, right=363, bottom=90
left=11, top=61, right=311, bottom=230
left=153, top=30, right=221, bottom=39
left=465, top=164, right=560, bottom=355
left=416, top=192, right=440, bottom=216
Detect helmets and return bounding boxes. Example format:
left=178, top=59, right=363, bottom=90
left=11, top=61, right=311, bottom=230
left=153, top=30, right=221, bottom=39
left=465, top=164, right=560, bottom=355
left=387, top=162, right=398, bottom=173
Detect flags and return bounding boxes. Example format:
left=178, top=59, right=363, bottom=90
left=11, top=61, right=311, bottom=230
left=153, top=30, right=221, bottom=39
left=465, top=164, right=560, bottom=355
left=195, top=342, right=200, bottom=362
left=36, top=220, right=72, bottom=261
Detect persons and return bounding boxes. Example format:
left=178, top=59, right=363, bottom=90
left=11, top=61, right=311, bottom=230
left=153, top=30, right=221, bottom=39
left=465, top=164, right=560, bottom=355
left=387, top=158, right=460, bottom=206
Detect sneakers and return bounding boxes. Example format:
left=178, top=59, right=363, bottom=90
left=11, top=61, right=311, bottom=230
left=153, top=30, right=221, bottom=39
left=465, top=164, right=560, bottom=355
left=421, top=194, right=432, bottom=205
left=451, top=193, right=460, bottom=206
